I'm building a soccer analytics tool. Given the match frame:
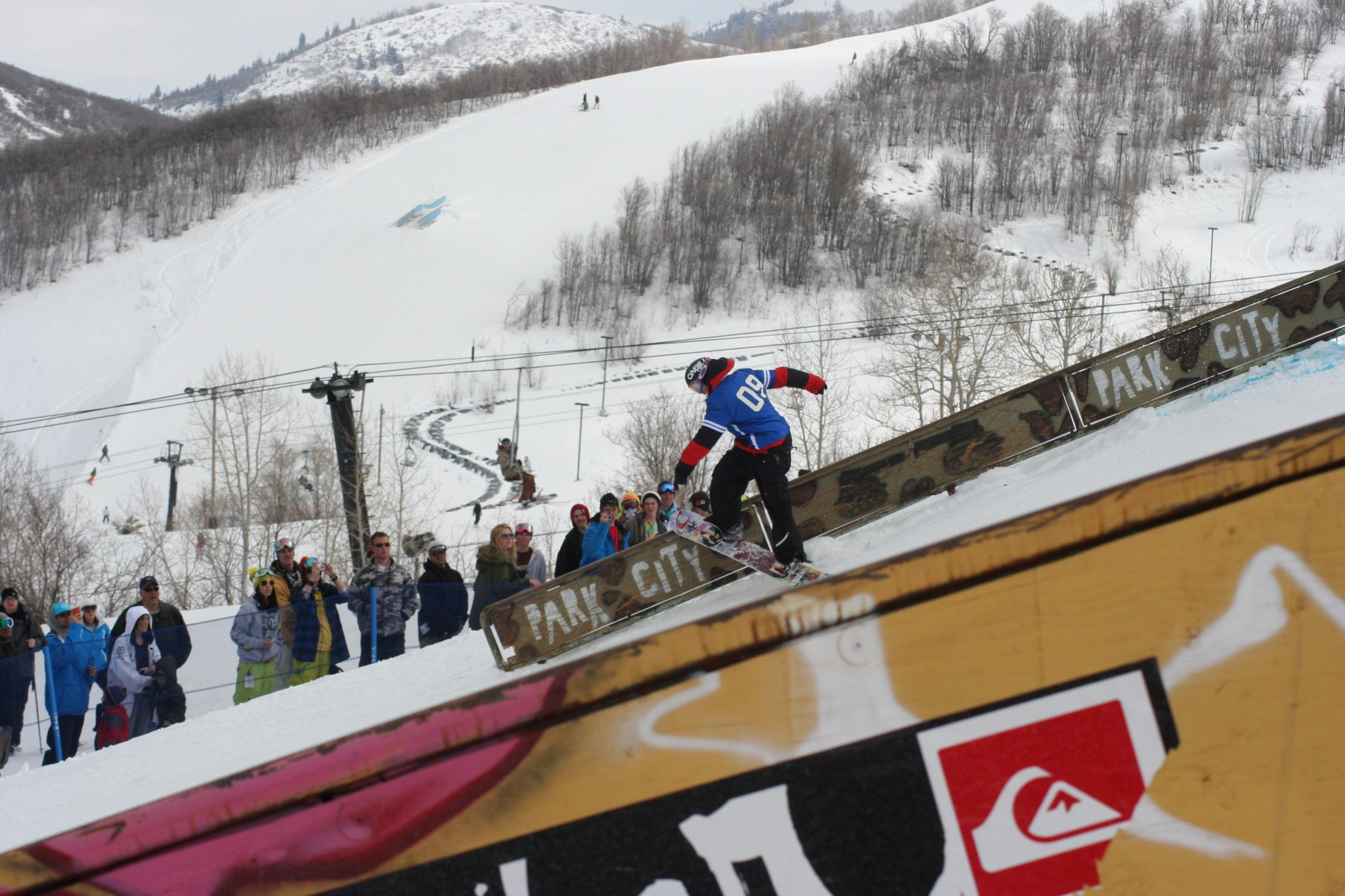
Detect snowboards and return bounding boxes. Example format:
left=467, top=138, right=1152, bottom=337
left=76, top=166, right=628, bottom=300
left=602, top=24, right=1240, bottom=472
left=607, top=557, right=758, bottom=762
left=515, top=494, right=557, bottom=510
left=669, top=509, right=832, bottom=589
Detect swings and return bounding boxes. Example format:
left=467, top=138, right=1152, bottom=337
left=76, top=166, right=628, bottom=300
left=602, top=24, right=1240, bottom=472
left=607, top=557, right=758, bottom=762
left=505, top=368, right=527, bottom=483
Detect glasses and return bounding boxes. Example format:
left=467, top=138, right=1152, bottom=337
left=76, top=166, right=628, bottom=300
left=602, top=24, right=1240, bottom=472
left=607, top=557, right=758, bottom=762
left=687, top=499, right=708, bottom=510
left=252, top=567, right=274, bottom=582
left=258, top=580, right=274, bottom=587
left=304, top=557, right=319, bottom=569
left=4, top=596, right=16, bottom=602
left=688, top=358, right=711, bottom=394
left=515, top=523, right=533, bottom=534
left=274, top=538, right=294, bottom=551
left=142, top=587, right=159, bottom=593
left=374, top=543, right=390, bottom=548
left=428, top=548, right=446, bottom=557
left=659, top=483, right=675, bottom=493
left=0, top=616, right=14, bottom=629
left=501, top=533, right=514, bottom=539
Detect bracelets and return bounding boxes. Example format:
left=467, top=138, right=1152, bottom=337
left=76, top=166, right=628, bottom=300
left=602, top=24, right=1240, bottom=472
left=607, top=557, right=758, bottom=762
left=330, top=572, right=338, bottom=582
left=306, top=579, right=314, bottom=587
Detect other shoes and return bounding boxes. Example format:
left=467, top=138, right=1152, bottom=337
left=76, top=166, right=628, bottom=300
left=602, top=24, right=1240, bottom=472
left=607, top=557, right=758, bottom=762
left=769, top=556, right=797, bottom=578
left=702, top=524, right=740, bottom=546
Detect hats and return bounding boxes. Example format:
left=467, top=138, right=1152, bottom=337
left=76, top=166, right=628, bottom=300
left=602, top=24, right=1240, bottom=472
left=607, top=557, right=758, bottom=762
left=600, top=493, right=619, bottom=509
left=426, top=540, right=447, bottom=551
left=249, top=567, right=276, bottom=589
left=53, top=601, right=72, bottom=616
left=690, top=490, right=711, bottom=509
left=79, top=598, right=97, bottom=609
left=139, top=576, right=158, bottom=589
left=684, top=358, right=709, bottom=386
left=570, top=504, right=590, bottom=524
left=0, top=587, right=19, bottom=600
left=104, top=684, right=128, bottom=706
left=621, top=480, right=676, bottom=508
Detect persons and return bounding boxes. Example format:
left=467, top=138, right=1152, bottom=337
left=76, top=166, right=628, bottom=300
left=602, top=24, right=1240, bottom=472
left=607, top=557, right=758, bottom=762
left=592, top=94, right=600, bottom=109
left=495, top=437, right=536, bottom=507
left=39, top=601, right=97, bottom=767
left=348, top=531, right=418, bottom=668
left=471, top=498, right=482, bottom=526
left=230, top=482, right=714, bottom=703
left=102, top=506, right=109, bottom=523
left=99, top=444, right=110, bottom=462
left=88, top=467, right=96, bottom=484
left=580, top=92, right=588, bottom=111
left=673, top=356, right=828, bottom=578
left=849, top=51, right=857, bottom=65
left=0, top=575, right=191, bottom=777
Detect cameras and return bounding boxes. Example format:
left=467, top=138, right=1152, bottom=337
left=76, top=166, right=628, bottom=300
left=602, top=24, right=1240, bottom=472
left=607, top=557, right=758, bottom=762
left=316, top=564, right=325, bottom=571
left=607, top=514, right=613, bottom=520
